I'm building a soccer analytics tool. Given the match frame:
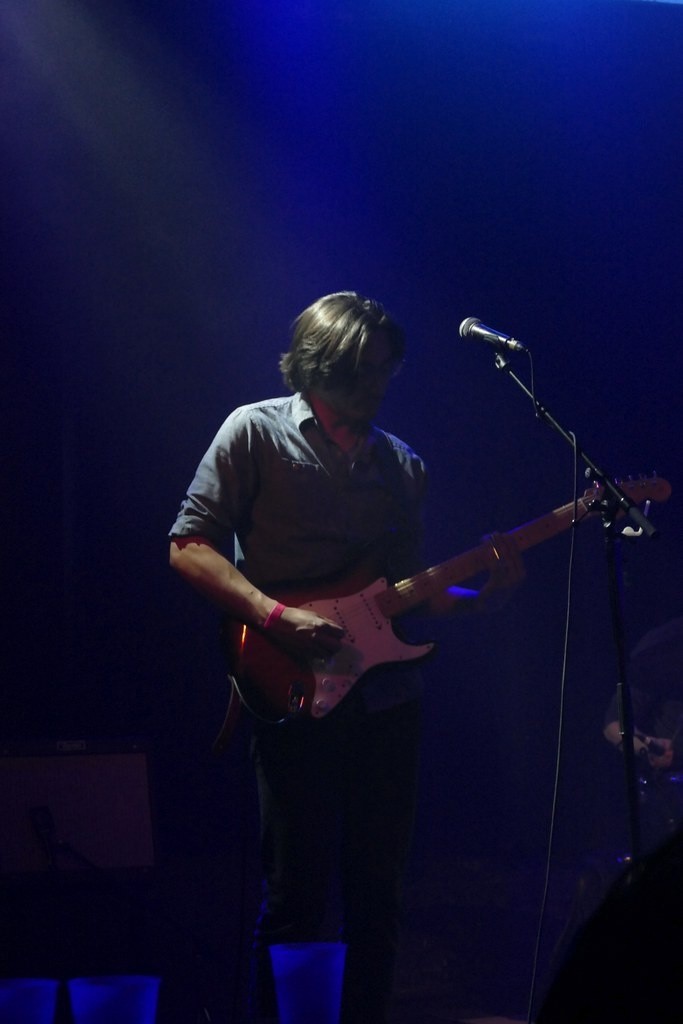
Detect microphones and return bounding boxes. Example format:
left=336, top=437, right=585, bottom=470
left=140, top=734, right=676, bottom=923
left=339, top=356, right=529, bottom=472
left=458, top=316, right=528, bottom=354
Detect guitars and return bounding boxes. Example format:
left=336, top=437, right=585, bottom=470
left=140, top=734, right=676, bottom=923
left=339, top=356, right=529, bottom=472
left=213, top=467, right=674, bottom=727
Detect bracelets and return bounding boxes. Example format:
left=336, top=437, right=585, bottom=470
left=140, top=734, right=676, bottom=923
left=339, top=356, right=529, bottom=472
left=263, top=603, right=286, bottom=631
left=616, top=740, right=623, bottom=747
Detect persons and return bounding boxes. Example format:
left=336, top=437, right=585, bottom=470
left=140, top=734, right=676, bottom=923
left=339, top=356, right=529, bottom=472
left=604, top=619, right=683, bottom=773
left=166, top=291, right=527, bottom=1023
